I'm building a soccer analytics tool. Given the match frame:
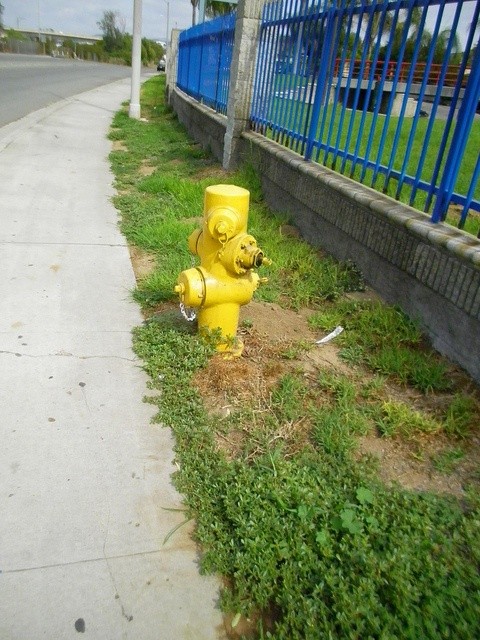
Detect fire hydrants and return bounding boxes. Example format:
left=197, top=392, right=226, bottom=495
left=174, top=185, right=272, bottom=361
